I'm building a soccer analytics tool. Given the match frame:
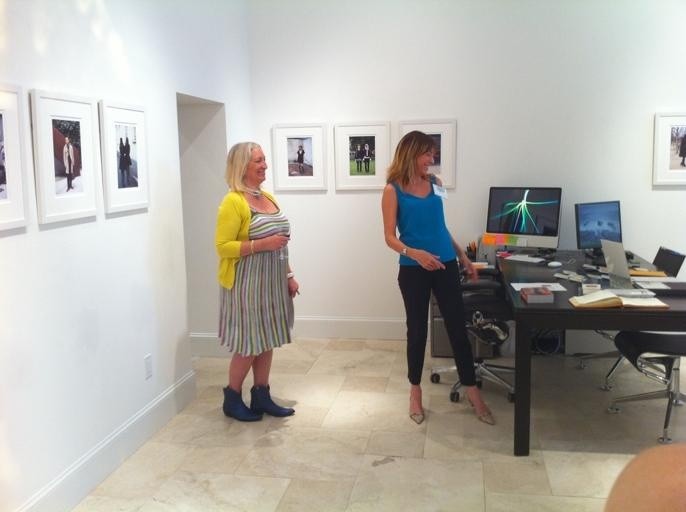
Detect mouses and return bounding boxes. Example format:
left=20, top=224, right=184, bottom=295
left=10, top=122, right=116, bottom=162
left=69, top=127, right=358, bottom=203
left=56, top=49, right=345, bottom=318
left=548, top=261, right=562, bottom=267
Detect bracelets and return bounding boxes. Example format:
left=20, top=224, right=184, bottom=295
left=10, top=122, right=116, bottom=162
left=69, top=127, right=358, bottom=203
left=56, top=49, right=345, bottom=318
left=249, top=240, right=255, bottom=255
left=286, top=272, right=294, bottom=280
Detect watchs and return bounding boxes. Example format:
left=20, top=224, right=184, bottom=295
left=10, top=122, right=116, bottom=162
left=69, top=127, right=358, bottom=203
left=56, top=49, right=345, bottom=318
left=400, top=246, right=408, bottom=257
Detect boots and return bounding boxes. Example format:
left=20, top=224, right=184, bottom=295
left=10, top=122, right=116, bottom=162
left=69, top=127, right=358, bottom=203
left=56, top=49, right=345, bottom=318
left=250, top=383, right=295, bottom=418
left=223, top=386, right=261, bottom=422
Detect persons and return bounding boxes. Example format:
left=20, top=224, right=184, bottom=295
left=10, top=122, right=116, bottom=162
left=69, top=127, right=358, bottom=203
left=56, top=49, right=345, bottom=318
left=120, top=138, right=133, bottom=185
left=361, top=143, right=371, bottom=171
left=213, top=142, right=299, bottom=421
left=297, top=146, right=304, bottom=172
left=354, top=143, right=363, bottom=172
left=381, top=131, right=495, bottom=426
left=64, top=136, right=74, bottom=190
left=678, top=134, right=686, bottom=168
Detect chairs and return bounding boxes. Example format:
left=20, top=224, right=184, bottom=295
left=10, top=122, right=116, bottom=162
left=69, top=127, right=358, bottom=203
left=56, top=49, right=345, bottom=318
left=430, top=255, right=514, bottom=401
left=606, top=329, right=686, bottom=444
left=573, top=245, right=686, bottom=390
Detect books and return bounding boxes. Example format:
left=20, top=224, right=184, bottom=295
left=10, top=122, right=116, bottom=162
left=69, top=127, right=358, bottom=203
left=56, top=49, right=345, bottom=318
left=521, top=287, right=553, bottom=303
left=569, top=290, right=668, bottom=308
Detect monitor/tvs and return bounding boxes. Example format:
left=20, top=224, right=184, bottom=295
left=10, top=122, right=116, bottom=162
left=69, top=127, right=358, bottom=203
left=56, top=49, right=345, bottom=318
left=481, top=186, right=563, bottom=256
left=574, top=201, right=622, bottom=258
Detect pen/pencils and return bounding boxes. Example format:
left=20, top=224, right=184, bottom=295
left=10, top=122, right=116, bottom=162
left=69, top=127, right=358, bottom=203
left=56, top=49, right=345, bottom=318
left=467, top=241, right=477, bottom=250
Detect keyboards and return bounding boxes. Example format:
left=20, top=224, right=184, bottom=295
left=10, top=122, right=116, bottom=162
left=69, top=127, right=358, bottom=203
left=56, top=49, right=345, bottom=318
left=504, top=254, right=546, bottom=263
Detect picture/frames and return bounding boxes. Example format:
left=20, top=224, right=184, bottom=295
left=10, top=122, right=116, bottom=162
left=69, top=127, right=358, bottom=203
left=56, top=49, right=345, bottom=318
left=28, top=88, right=98, bottom=226
left=398, top=123, right=456, bottom=189
left=271, top=125, right=328, bottom=192
left=98, top=99, right=150, bottom=217
left=652, top=113, right=686, bottom=187
left=0, top=82, right=27, bottom=232
left=332, top=126, right=390, bottom=191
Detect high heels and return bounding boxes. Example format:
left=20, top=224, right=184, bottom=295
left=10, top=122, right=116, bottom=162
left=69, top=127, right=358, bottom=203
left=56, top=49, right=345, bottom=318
left=467, top=393, right=498, bottom=426
left=406, top=392, right=427, bottom=426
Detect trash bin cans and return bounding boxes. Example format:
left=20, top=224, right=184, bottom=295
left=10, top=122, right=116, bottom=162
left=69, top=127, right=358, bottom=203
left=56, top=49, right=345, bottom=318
left=466, top=325, right=494, bottom=359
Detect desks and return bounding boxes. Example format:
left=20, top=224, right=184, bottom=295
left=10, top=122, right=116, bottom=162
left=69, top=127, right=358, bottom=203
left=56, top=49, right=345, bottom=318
left=497, top=249, right=686, bottom=456
left=606, top=443, right=686, bottom=512
